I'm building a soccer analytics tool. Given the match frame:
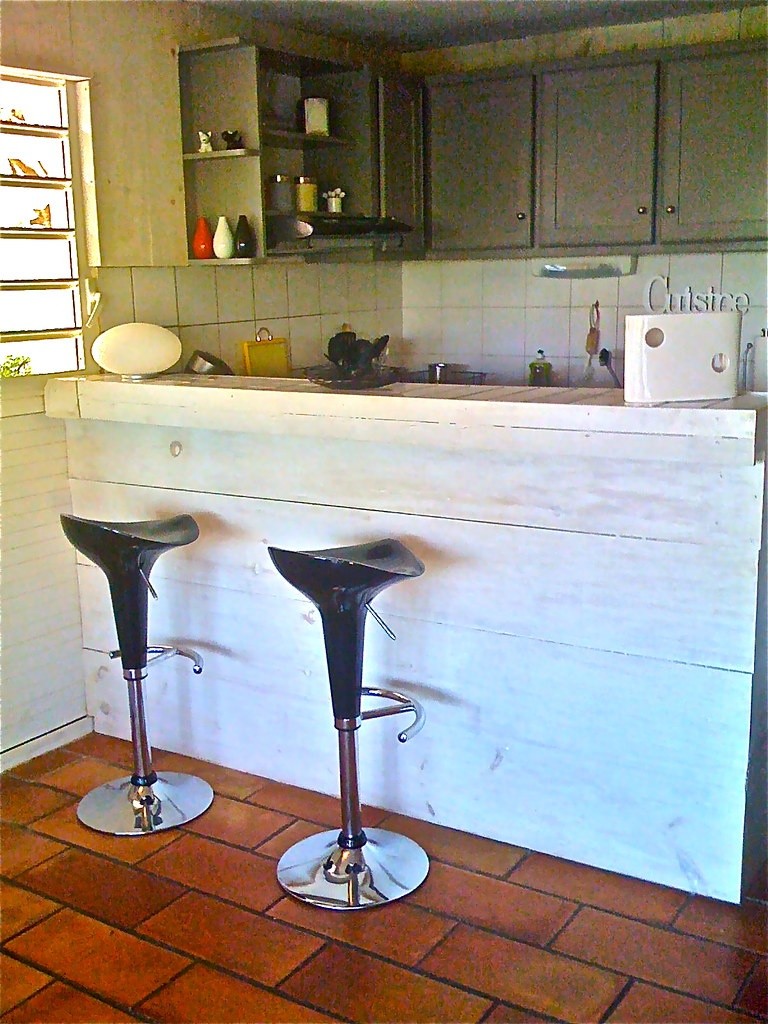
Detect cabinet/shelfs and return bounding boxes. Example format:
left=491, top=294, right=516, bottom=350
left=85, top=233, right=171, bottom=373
left=152, top=37, right=768, bottom=266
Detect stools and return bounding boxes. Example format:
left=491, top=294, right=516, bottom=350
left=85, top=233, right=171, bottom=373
left=61, top=512, right=215, bottom=838
left=268, top=538, right=430, bottom=911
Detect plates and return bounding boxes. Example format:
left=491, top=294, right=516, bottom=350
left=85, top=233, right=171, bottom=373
left=297, top=220, right=314, bottom=238
left=315, top=218, right=377, bottom=234
left=89, top=323, right=184, bottom=375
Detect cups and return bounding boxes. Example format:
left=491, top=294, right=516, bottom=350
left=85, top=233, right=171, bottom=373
left=270, top=183, right=295, bottom=211
left=428, top=363, right=446, bottom=382
left=305, top=98, right=329, bottom=136
left=295, top=183, right=319, bottom=212
left=328, top=198, right=341, bottom=213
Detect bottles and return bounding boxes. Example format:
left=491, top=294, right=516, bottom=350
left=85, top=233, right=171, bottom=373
left=529, top=351, right=553, bottom=387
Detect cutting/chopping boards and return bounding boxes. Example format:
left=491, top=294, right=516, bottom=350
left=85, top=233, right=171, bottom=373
left=242, top=327, right=292, bottom=379
